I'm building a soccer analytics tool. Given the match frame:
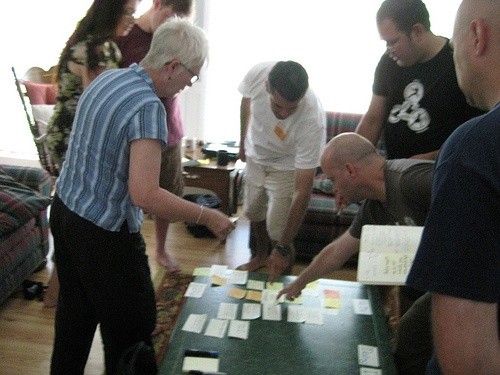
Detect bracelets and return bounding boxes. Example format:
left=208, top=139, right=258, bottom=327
left=195, top=205, right=205, bottom=224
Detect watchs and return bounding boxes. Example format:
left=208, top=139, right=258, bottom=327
left=275, top=244, right=292, bottom=256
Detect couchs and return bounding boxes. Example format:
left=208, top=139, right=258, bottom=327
left=229, top=111, right=361, bottom=258
left=0, top=164, right=53, bottom=305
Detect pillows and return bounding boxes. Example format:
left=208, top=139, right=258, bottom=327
left=0, top=169, right=52, bottom=236
left=312, top=169, right=334, bottom=195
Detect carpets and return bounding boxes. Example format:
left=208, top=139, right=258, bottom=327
left=153, top=268, right=195, bottom=367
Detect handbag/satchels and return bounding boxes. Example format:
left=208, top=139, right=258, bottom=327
left=186, top=194, right=224, bottom=239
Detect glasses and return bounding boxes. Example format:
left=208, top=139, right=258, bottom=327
left=165, top=61, right=199, bottom=85
left=123, top=9, right=133, bottom=18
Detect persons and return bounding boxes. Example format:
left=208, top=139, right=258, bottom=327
left=118, top=0, right=193, bottom=272
left=48, top=16, right=238, bottom=375
left=47, top=0, right=142, bottom=262
left=406, top=0, right=500, bottom=375
left=276, top=132, right=444, bottom=375
left=354, top=0, right=489, bottom=160
left=234, top=60, right=327, bottom=277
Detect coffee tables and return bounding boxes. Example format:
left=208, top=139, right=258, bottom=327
left=161, top=269, right=398, bottom=375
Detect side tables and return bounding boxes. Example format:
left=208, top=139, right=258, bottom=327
left=184, top=146, right=237, bottom=211
